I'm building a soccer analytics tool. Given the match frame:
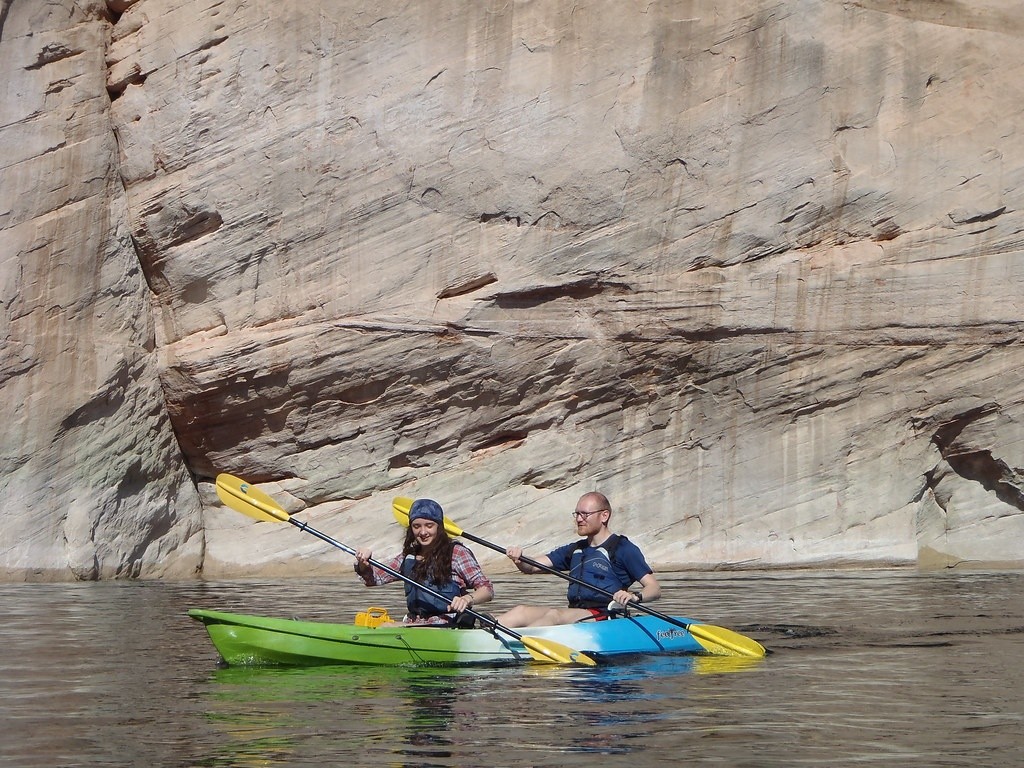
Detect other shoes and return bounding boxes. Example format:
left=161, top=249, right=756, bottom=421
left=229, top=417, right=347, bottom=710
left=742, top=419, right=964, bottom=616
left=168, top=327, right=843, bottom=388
left=479, top=613, right=497, bottom=628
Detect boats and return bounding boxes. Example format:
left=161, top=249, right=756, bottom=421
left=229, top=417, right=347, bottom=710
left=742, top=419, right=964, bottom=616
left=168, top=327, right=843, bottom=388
left=187, top=609, right=769, bottom=667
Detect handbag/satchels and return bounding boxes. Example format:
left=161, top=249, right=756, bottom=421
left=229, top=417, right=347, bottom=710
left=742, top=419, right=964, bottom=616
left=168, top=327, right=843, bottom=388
left=354, top=608, right=395, bottom=628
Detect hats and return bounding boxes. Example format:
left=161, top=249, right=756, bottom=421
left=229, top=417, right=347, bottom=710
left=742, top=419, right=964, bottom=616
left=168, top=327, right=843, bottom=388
left=409, top=499, right=443, bottom=526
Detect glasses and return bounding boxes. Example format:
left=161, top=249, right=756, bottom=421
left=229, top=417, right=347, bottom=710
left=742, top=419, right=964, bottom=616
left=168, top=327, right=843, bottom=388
left=572, top=509, right=604, bottom=520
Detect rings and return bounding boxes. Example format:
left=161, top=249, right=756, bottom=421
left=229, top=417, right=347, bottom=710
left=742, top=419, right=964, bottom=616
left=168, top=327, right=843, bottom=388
left=509, top=550, right=513, bottom=553
left=358, top=552, right=362, bottom=554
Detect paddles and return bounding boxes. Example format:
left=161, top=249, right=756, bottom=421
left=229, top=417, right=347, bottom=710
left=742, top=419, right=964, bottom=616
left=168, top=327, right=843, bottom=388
left=391, top=497, right=769, bottom=660
left=216, top=472, right=599, bottom=667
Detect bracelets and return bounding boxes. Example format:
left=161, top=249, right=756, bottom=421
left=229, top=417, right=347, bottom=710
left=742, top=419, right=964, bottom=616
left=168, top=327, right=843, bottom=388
left=632, top=592, right=642, bottom=604
left=466, top=593, right=476, bottom=605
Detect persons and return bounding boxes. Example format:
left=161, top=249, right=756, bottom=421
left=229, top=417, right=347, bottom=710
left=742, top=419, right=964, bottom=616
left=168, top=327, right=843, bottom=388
left=476, top=491, right=662, bottom=630
left=354, top=498, right=495, bottom=630
